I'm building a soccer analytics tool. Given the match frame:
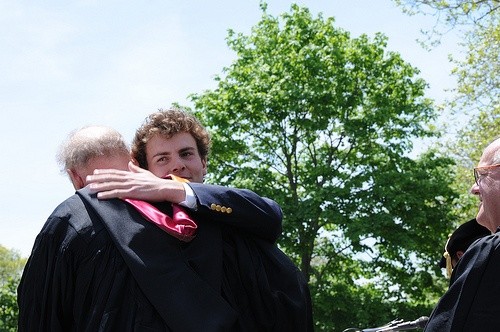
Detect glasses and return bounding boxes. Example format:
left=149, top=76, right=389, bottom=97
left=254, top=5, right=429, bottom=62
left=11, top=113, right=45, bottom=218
left=473, top=163, right=500, bottom=186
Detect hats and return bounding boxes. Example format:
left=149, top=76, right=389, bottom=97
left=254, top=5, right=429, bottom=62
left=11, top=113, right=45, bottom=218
left=439, top=218, right=492, bottom=268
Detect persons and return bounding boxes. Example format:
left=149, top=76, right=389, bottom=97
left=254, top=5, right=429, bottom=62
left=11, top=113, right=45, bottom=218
left=16, top=108, right=313, bottom=332
left=422, top=136, right=500, bottom=332
